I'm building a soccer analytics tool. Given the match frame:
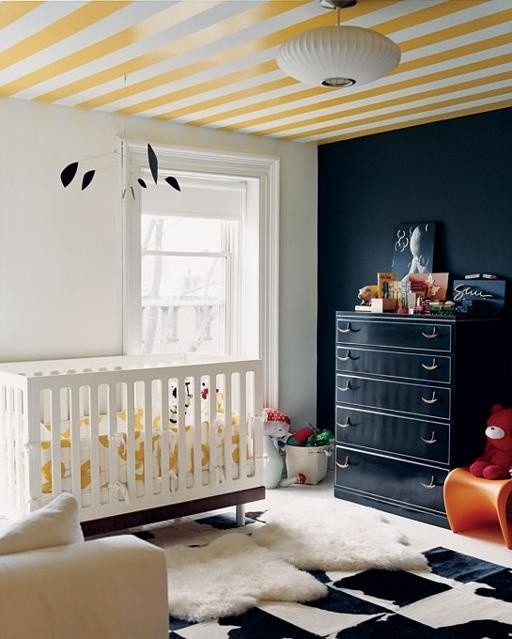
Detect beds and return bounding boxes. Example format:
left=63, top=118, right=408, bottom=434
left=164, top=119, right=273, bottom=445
left=0, top=355, right=267, bottom=537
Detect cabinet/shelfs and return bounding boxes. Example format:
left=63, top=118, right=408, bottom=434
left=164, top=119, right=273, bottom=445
left=334, top=310, right=512, bottom=530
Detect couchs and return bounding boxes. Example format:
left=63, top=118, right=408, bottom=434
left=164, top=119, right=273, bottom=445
left=0, top=532, right=169, bottom=639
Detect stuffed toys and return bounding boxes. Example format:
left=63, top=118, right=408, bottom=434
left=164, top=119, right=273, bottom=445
left=264, top=409, right=313, bottom=448
left=166, top=381, right=194, bottom=424
left=469, top=403, right=511, bottom=481
left=201, top=382, right=224, bottom=418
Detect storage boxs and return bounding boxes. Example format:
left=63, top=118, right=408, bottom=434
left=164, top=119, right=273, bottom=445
left=280, top=445, right=334, bottom=484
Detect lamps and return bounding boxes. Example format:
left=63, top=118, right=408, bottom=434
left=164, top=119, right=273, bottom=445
left=274, top=0, right=401, bottom=91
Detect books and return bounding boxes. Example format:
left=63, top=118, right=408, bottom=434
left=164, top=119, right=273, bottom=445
left=384, top=281, right=427, bottom=308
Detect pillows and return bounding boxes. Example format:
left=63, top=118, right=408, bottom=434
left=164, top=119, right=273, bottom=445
left=1, top=492, right=84, bottom=555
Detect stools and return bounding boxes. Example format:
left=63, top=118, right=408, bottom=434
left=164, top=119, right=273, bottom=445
left=442, top=465, right=510, bottom=548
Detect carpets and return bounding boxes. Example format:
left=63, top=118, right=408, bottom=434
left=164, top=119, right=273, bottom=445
left=158, top=531, right=326, bottom=622
left=248, top=501, right=433, bottom=574
